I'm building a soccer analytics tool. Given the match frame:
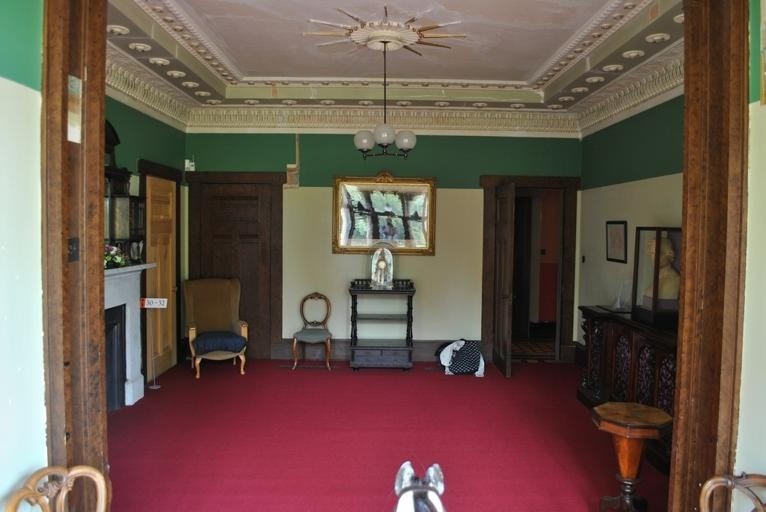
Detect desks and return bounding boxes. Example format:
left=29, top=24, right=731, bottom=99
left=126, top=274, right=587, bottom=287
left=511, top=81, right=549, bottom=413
left=347, top=279, right=416, bottom=372
left=591, top=401, right=672, bottom=512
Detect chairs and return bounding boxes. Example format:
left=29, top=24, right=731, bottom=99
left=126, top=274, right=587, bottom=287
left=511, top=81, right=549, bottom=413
left=289, top=292, right=332, bottom=372
left=699, top=472, right=766, bottom=512
left=5, top=465, right=108, bottom=512
left=184, top=276, right=249, bottom=379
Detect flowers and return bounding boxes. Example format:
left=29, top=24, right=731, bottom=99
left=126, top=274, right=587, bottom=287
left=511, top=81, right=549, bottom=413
left=104, top=242, right=132, bottom=269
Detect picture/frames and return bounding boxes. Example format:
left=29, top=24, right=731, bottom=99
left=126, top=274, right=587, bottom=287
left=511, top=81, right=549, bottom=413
left=605, top=221, right=627, bottom=264
left=332, top=171, right=437, bottom=257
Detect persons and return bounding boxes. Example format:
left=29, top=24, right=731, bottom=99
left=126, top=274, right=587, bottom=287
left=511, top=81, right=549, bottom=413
left=643, top=237, right=681, bottom=298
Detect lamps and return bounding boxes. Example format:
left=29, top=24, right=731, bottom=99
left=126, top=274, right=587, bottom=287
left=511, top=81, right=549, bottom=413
left=354, top=39, right=416, bottom=159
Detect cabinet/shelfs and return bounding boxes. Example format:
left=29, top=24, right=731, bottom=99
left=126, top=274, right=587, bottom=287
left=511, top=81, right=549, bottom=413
left=630, top=226, right=682, bottom=333
left=577, top=305, right=678, bottom=474
